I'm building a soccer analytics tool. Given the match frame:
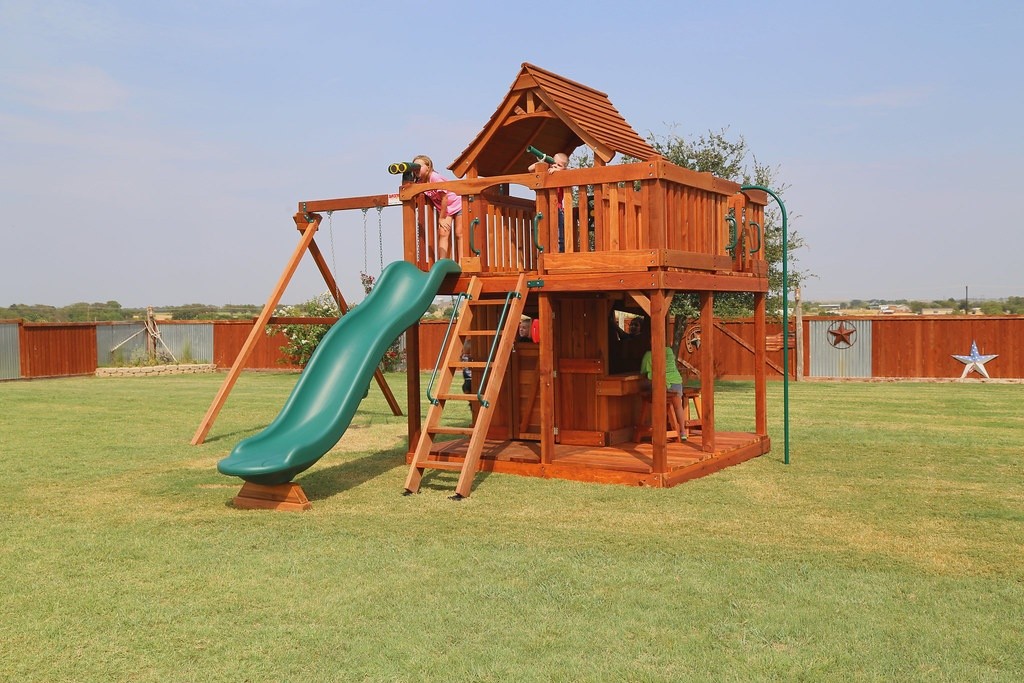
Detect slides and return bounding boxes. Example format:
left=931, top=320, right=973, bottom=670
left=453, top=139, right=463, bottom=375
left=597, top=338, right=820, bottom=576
left=215, top=260, right=463, bottom=488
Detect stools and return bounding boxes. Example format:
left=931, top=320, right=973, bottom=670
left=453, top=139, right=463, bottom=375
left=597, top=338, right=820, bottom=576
left=632, top=389, right=681, bottom=443
left=682, top=387, right=701, bottom=438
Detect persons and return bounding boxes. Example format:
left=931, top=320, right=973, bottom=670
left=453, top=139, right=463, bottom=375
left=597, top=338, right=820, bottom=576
left=528, top=151, right=570, bottom=252
left=515, top=318, right=532, bottom=342
left=413, top=155, right=464, bottom=267
left=615, top=317, right=644, bottom=341
left=640, top=347, right=687, bottom=440
left=459, top=340, right=472, bottom=412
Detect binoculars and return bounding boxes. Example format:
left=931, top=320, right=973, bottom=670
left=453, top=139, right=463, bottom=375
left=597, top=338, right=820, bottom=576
left=388, top=162, right=421, bottom=174
